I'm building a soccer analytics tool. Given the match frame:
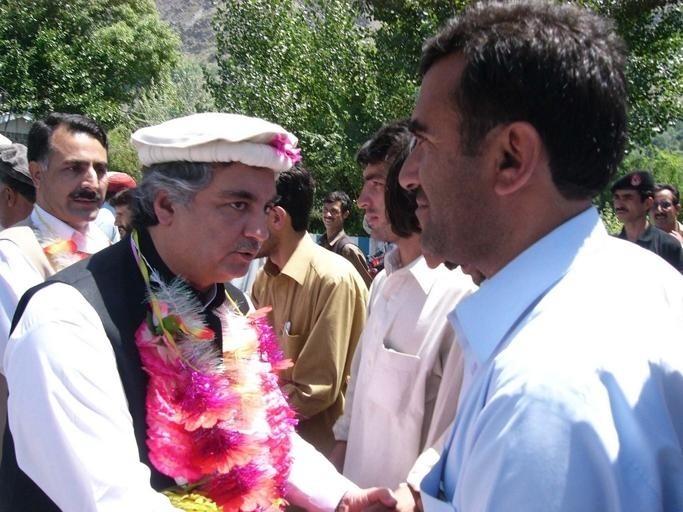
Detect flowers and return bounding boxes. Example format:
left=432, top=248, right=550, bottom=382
left=135, top=292, right=301, bottom=512
left=268, top=133, right=307, bottom=165
left=40, top=232, right=89, bottom=264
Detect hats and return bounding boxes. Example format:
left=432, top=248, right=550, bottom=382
left=132, top=113, right=298, bottom=173
left=107, top=174, right=136, bottom=193
left=0, top=143, right=34, bottom=187
left=611, top=171, right=654, bottom=193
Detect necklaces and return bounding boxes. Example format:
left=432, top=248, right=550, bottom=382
left=129, top=225, right=256, bottom=384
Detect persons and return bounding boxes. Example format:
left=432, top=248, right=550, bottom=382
left=361, top=0, right=683, bottom=511
left=326, top=120, right=479, bottom=498
left=611, top=171, right=683, bottom=274
left=3, top=111, right=399, bottom=510
left=320, top=191, right=376, bottom=287
left=2, top=111, right=137, bottom=431
left=247, top=165, right=374, bottom=476
